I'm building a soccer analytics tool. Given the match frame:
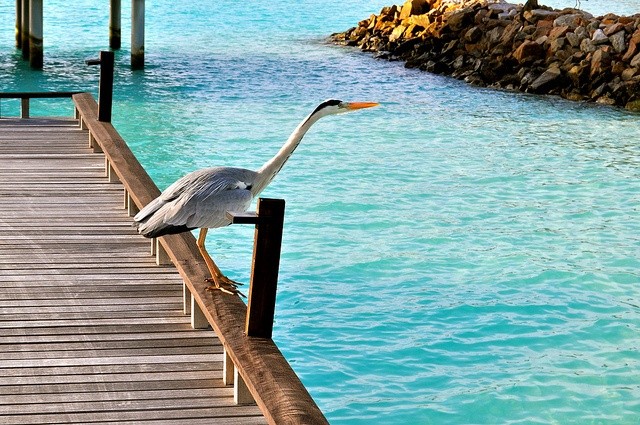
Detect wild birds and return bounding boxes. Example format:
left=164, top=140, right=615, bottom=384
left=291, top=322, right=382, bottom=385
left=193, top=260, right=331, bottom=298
left=130, top=96, right=382, bottom=299
